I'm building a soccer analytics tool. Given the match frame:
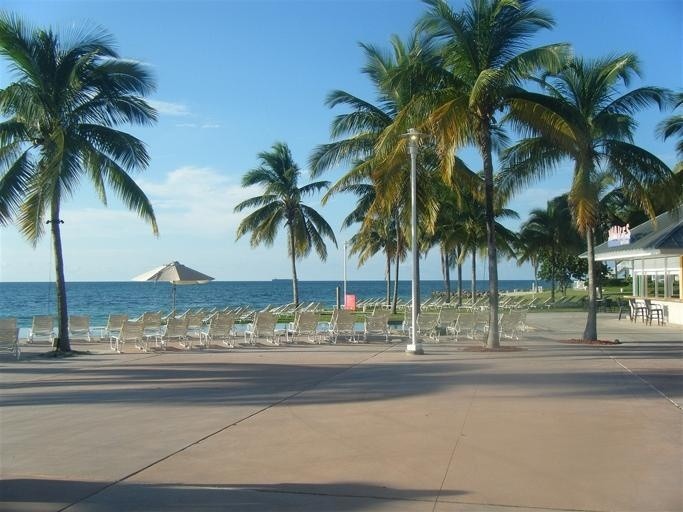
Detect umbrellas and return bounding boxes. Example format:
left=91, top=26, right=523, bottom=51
left=131, top=261, right=215, bottom=318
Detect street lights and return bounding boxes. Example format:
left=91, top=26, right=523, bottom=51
left=341, top=238, right=349, bottom=305
left=401, top=128, right=426, bottom=356
left=42, top=219, right=65, bottom=317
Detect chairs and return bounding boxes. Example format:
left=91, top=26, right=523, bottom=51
left=0, top=293, right=664, bottom=360
